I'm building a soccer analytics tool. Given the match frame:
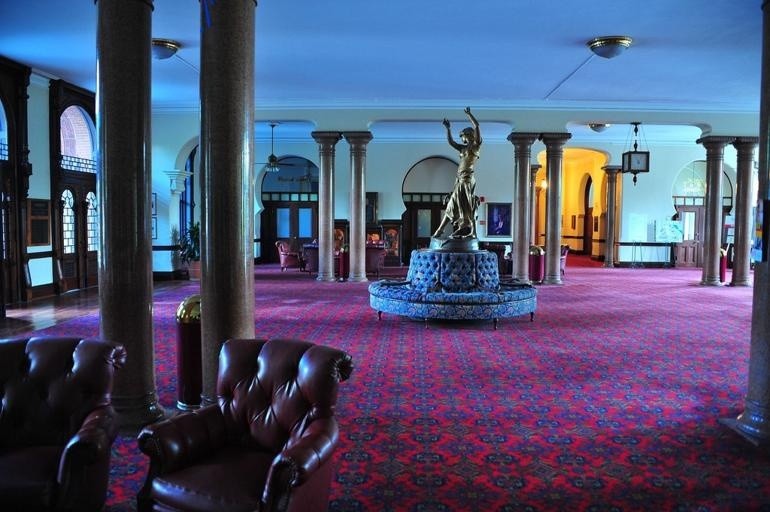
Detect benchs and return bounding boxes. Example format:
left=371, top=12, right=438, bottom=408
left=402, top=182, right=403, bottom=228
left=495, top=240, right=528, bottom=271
left=368, top=253, right=537, bottom=330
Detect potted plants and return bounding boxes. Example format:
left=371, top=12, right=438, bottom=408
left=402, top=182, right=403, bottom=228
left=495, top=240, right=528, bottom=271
left=177, top=222, right=203, bottom=282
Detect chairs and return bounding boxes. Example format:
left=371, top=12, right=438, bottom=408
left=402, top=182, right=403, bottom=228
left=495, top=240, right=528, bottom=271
left=133, top=338, right=353, bottom=512
left=480, top=241, right=569, bottom=283
left=275, top=239, right=390, bottom=277
left=0, top=336, right=127, bottom=512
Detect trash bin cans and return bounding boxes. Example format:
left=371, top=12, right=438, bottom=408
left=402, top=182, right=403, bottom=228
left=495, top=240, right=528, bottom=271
left=177, top=294, right=203, bottom=412
left=720, top=248, right=726, bottom=282
left=339, top=244, right=349, bottom=281
left=529, top=245, right=545, bottom=285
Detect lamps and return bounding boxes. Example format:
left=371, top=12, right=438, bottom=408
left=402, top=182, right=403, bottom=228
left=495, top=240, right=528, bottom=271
left=622, top=121, right=648, bottom=186
left=586, top=36, right=632, bottom=59
left=541, top=178, right=547, bottom=189
left=265, top=162, right=280, bottom=174
left=151, top=37, right=181, bottom=61
left=589, top=124, right=610, bottom=134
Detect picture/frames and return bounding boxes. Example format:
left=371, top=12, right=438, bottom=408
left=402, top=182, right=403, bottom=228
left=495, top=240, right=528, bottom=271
left=484, top=202, right=513, bottom=238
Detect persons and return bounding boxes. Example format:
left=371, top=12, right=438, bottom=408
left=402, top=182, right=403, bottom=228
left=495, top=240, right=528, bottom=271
left=434, top=107, right=483, bottom=239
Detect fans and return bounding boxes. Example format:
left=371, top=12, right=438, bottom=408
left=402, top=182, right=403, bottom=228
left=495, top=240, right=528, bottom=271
left=254, top=122, right=297, bottom=168
